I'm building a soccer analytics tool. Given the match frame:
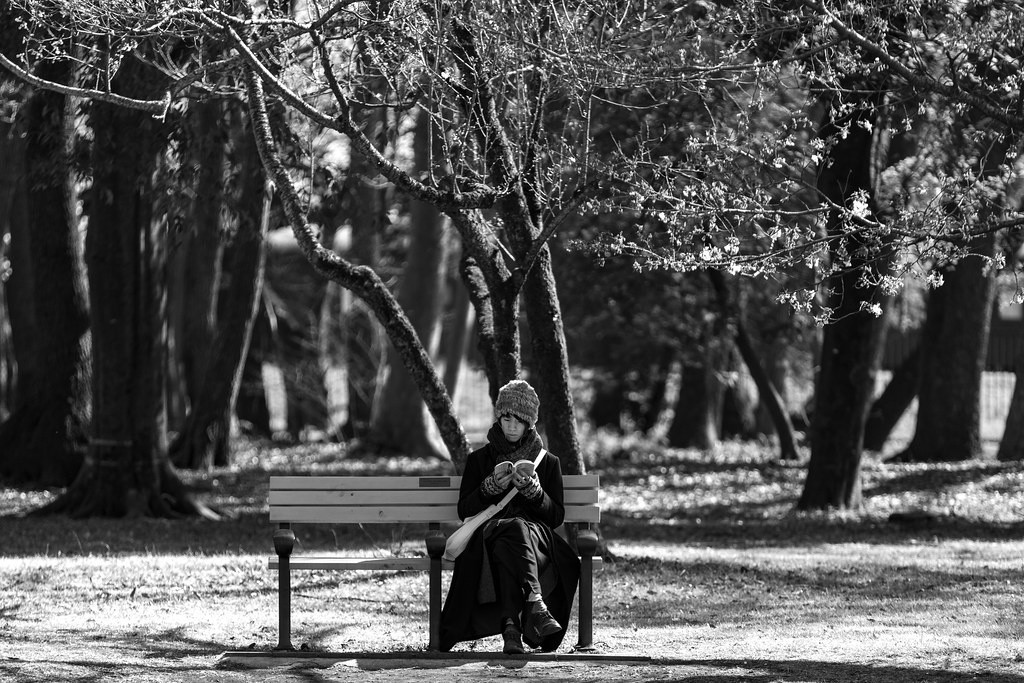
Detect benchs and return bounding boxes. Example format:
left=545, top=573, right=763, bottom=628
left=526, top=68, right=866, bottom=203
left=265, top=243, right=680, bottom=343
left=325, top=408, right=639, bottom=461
left=266, top=475, right=600, bottom=652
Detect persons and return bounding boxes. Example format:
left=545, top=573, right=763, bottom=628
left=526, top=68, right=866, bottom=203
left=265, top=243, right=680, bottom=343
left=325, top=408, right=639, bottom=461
left=506, top=463, right=512, bottom=474
left=439, top=380, right=582, bottom=653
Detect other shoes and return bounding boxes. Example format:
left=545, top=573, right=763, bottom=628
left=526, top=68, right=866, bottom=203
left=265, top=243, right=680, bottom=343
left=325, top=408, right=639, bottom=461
left=531, top=608, right=562, bottom=638
left=502, top=626, right=525, bottom=654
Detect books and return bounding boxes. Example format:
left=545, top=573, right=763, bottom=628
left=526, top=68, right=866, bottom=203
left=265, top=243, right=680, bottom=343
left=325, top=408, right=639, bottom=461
left=494, top=460, right=534, bottom=479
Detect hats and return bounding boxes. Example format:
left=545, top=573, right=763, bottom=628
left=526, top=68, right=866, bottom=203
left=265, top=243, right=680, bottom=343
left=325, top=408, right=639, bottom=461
left=494, top=379, right=540, bottom=429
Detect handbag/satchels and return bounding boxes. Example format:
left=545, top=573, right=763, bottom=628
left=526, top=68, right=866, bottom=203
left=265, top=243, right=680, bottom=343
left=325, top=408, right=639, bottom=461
left=441, top=503, right=500, bottom=562
